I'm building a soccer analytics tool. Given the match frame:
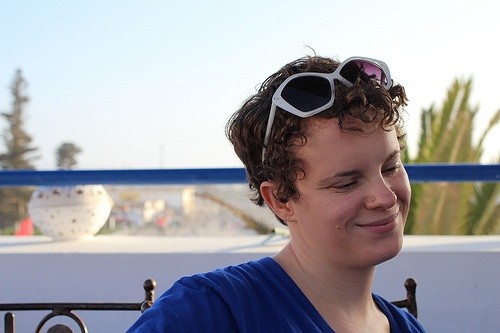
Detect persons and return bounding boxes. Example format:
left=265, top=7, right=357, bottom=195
left=125, top=55, right=428, bottom=333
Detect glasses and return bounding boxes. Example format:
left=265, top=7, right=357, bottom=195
left=261, top=56, right=391, bottom=182
